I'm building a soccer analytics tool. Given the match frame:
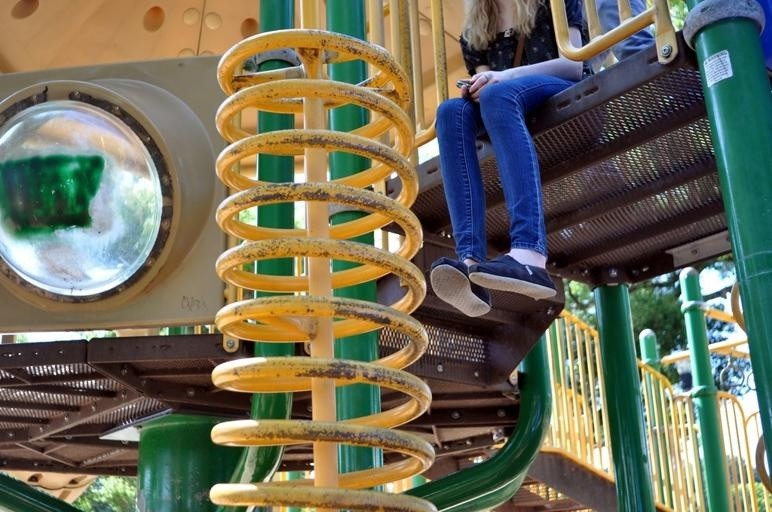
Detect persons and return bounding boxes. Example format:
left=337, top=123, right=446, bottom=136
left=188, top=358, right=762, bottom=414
left=590, top=0, right=702, bottom=113
left=429, top=0, right=604, bottom=318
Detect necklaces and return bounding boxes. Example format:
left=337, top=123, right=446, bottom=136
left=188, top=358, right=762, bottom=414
left=500, top=23, right=519, bottom=38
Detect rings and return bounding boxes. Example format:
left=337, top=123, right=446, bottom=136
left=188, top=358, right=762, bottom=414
left=484, top=73, right=489, bottom=81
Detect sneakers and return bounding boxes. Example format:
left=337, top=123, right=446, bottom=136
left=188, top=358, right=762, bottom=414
left=468, top=255, right=557, bottom=299
left=430, top=257, right=492, bottom=317
